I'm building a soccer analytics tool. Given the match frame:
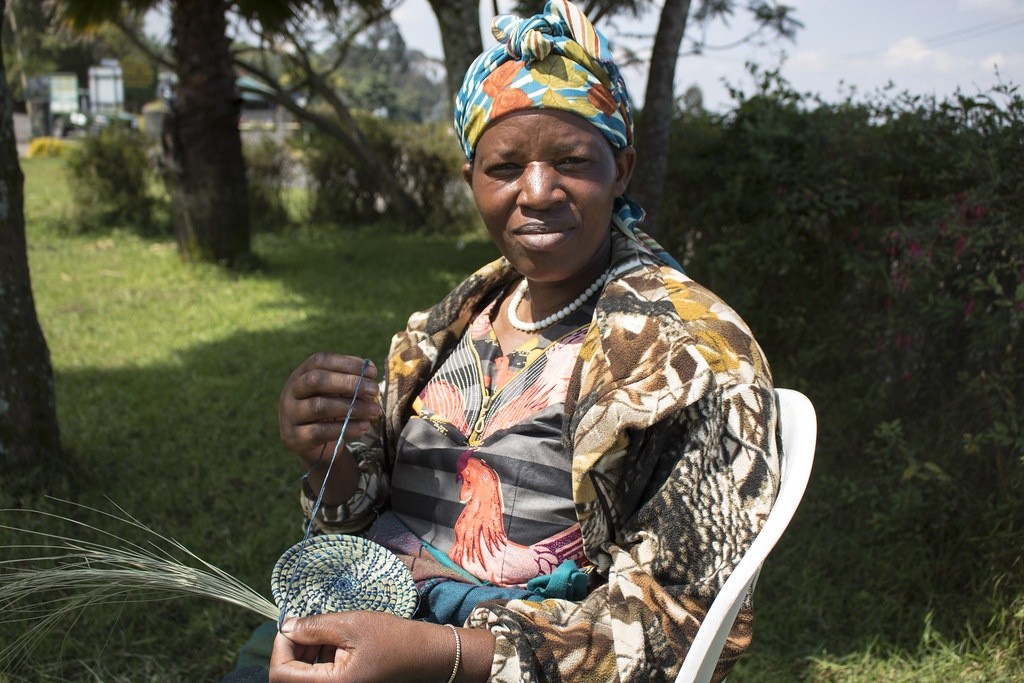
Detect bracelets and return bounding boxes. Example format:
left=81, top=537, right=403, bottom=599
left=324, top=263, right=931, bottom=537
left=444, top=624, right=461, bottom=683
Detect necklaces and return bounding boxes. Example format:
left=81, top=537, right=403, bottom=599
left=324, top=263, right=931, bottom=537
left=507, top=269, right=609, bottom=332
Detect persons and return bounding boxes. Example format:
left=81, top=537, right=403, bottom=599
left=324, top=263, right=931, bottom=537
left=268, top=0, right=786, bottom=683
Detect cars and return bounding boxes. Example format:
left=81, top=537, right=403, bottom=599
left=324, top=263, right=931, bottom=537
left=160, top=72, right=307, bottom=122
